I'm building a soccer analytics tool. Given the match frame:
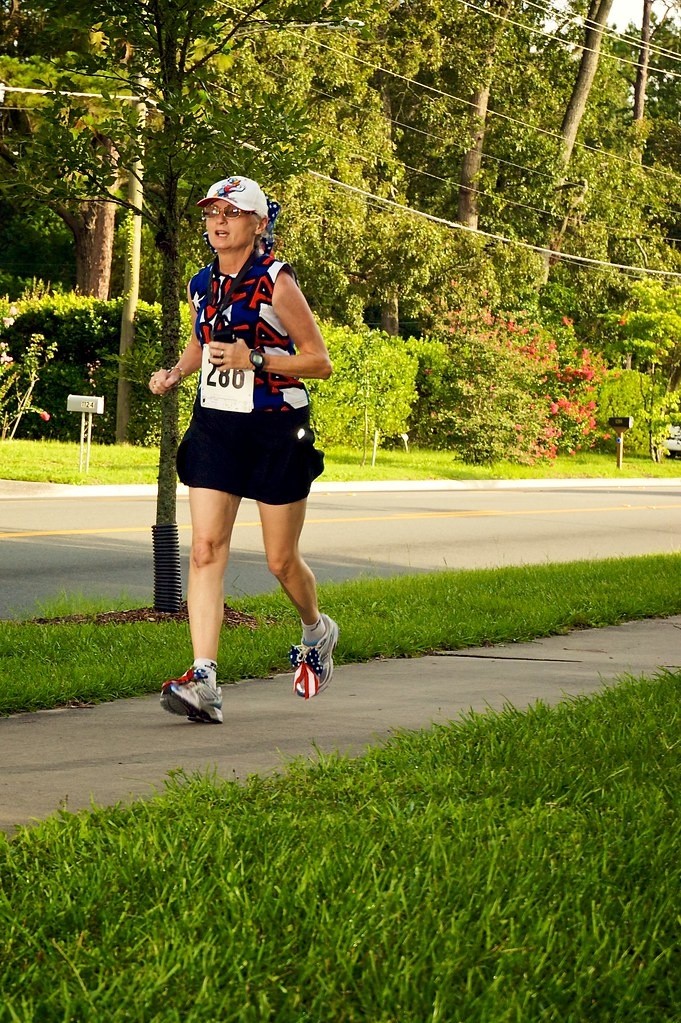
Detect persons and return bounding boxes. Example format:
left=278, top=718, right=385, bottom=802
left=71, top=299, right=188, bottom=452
left=148, top=176, right=338, bottom=725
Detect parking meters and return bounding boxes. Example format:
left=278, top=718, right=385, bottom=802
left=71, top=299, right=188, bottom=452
left=609, top=416, right=634, bottom=469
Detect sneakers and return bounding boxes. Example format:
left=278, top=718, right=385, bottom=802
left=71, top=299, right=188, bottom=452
left=160, top=666, right=224, bottom=723
left=288, top=613, right=338, bottom=700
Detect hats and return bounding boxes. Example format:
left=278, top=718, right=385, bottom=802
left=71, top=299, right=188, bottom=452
left=197, top=176, right=268, bottom=219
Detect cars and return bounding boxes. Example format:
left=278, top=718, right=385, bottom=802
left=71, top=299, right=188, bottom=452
left=662, top=426, right=681, bottom=459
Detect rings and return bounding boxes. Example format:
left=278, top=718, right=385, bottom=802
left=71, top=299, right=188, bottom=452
left=221, top=351, right=223, bottom=357
left=221, top=359, right=223, bottom=364
left=154, top=381, right=156, bottom=384
left=153, top=385, right=155, bottom=388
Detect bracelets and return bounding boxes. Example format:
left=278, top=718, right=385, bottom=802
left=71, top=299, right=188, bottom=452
left=168, top=367, right=184, bottom=386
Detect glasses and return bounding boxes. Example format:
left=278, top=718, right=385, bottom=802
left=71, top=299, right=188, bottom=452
left=201, top=204, right=257, bottom=219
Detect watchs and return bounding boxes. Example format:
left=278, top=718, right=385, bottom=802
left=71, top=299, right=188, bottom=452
left=249, top=348, right=265, bottom=372
